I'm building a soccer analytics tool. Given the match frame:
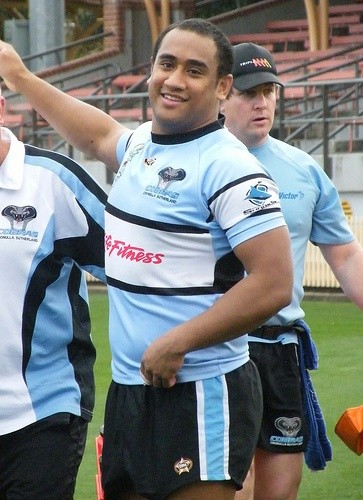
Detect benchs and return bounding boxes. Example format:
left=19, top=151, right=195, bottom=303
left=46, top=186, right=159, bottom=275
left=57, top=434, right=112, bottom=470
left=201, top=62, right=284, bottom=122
left=1, top=2, right=363, bottom=161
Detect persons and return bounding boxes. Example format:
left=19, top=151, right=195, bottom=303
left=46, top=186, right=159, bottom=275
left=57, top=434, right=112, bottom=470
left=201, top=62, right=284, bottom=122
left=217, top=41, right=362, bottom=499
left=1, top=19, right=294, bottom=500
left=0, top=94, right=109, bottom=499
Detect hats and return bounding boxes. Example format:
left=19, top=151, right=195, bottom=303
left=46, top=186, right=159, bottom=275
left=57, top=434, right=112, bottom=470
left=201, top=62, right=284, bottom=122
left=231, top=42, right=285, bottom=92
